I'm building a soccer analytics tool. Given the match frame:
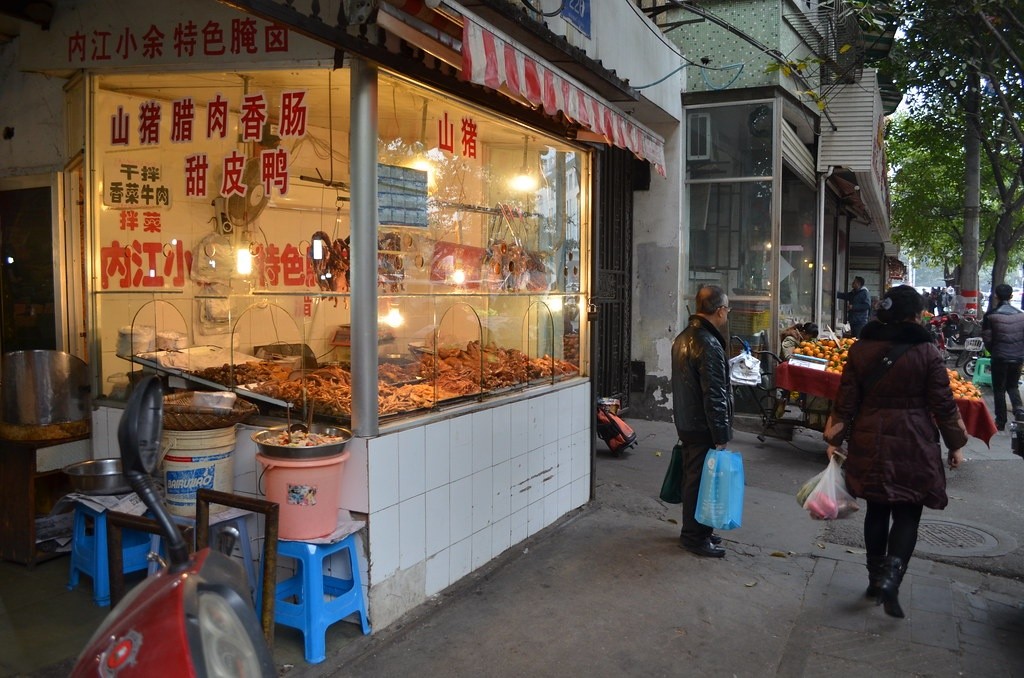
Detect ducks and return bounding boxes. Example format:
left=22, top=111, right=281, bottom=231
left=485, top=238, right=555, bottom=294
left=310, top=231, right=406, bottom=311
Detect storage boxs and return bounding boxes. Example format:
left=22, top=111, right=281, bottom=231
left=728, top=310, right=776, bottom=414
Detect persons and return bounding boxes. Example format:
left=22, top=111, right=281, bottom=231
left=919, top=287, right=959, bottom=318
left=981, top=283, right=1024, bottom=432
left=671, top=283, right=734, bottom=558
left=824, top=285, right=968, bottom=619
left=834, top=276, right=872, bottom=338
left=780, top=322, right=819, bottom=361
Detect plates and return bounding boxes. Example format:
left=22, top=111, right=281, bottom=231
left=158, top=332, right=187, bottom=350
left=117, top=325, right=152, bottom=356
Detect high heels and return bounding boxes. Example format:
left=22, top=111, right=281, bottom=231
left=865, top=556, right=906, bottom=618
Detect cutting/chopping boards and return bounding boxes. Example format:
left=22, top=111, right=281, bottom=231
left=0, top=422, right=91, bottom=439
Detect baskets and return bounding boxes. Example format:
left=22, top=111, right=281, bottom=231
left=965, top=337, right=984, bottom=352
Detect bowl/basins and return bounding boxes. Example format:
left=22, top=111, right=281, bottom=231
left=383, top=351, right=407, bottom=363
left=63, top=458, right=135, bottom=495
left=251, top=423, right=355, bottom=458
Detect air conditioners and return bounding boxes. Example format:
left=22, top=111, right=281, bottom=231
left=687, top=112, right=714, bottom=163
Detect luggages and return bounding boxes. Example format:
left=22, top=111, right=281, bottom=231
left=596, top=401, right=638, bottom=456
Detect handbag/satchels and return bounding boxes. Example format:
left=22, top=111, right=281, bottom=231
left=796, top=455, right=861, bottom=520
left=659, top=444, right=686, bottom=504
left=695, top=448, right=744, bottom=531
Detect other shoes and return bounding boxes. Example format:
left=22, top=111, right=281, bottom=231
left=1014, top=410, right=1024, bottom=421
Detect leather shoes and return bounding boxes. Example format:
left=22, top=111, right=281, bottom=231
left=679, top=533, right=726, bottom=557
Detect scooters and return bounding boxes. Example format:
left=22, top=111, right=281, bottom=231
left=66, top=373, right=278, bottom=678
left=928, top=313, right=990, bottom=377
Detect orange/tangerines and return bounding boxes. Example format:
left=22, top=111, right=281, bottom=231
left=792, top=338, right=856, bottom=373
left=947, top=368, right=982, bottom=399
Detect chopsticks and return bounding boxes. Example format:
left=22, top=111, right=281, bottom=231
left=308, top=396, right=315, bottom=432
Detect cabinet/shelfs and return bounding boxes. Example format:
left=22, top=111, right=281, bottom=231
left=0, top=434, right=90, bottom=570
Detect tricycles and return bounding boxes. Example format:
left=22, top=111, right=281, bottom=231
left=728, top=333, right=853, bottom=449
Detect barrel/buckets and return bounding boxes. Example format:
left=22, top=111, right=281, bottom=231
left=155, top=425, right=236, bottom=516
left=256, top=448, right=355, bottom=540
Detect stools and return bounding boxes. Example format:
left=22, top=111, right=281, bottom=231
left=68, top=505, right=371, bottom=663
left=971, top=357, right=992, bottom=386
